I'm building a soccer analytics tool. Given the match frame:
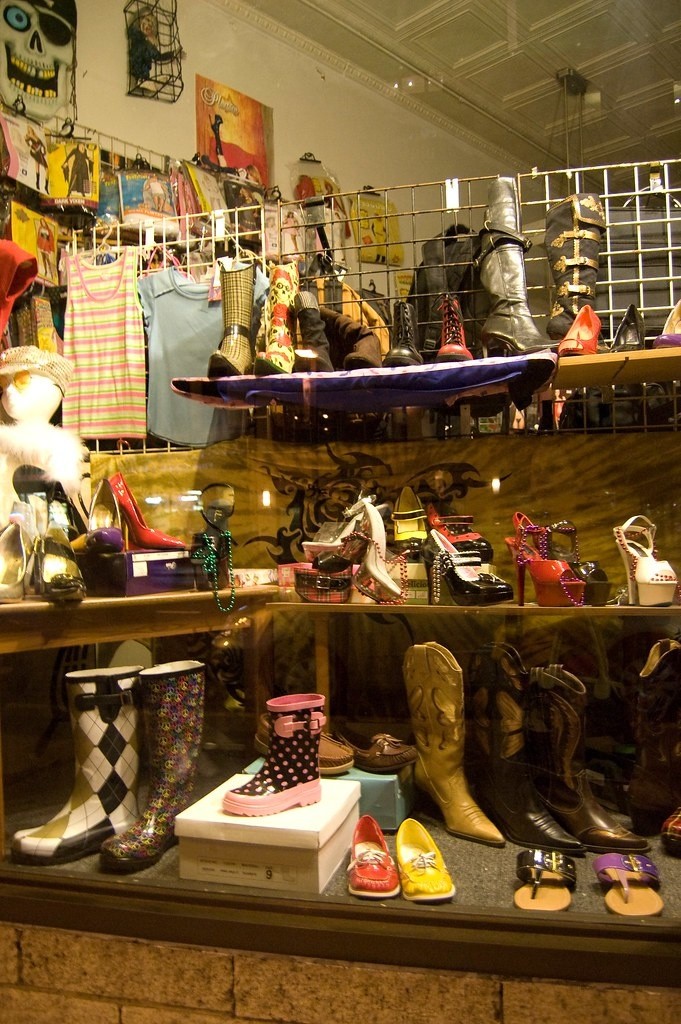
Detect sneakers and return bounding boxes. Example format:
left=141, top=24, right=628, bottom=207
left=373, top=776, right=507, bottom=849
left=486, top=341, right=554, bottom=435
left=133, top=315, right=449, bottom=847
left=255, top=713, right=353, bottom=774
left=334, top=728, right=419, bottom=772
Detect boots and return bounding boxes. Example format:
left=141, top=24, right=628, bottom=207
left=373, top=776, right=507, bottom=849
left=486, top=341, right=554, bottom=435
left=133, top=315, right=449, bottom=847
left=10, top=664, right=142, bottom=865
left=628, top=637, right=681, bottom=836
left=526, top=662, right=652, bottom=852
left=463, top=639, right=586, bottom=851
left=224, top=693, right=328, bottom=816
left=100, top=660, right=205, bottom=865
left=402, top=641, right=505, bottom=845
left=205, top=175, right=681, bottom=376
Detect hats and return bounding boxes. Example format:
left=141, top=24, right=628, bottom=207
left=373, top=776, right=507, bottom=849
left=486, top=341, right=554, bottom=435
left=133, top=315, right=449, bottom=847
left=0, top=345, right=74, bottom=398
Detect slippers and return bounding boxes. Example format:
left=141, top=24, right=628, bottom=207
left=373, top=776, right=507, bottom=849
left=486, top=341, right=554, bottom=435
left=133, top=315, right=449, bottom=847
left=591, top=853, right=664, bottom=916
left=514, top=848, right=577, bottom=911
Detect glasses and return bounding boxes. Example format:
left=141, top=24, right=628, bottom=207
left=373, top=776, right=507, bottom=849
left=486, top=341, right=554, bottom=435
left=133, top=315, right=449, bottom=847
left=0, top=372, right=32, bottom=390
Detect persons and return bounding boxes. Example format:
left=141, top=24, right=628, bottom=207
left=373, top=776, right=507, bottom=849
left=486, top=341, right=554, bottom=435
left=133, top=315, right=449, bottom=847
left=144, top=176, right=168, bottom=212
left=282, top=212, right=301, bottom=252
left=0, top=345, right=87, bottom=528
left=37, top=218, right=56, bottom=279
left=210, top=114, right=262, bottom=239
left=24, top=126, right=49, bottom=194
left=63, top=143, right=89, bottom=198
left=370, top=214, right=386, bottom=263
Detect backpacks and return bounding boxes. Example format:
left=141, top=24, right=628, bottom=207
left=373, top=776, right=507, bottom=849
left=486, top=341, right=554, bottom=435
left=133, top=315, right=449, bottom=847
left=405, top=222, right=508, bottom=365
left=299, top=254, right=390, bottom=367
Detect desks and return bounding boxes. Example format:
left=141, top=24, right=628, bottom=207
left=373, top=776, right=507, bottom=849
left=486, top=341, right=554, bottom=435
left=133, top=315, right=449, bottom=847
left=0, top=584, right=280, bottom=765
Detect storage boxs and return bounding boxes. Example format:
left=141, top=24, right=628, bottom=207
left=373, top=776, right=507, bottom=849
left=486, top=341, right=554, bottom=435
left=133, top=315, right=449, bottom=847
left=379, top=563, right=452, bottom=605
left=173, top=755, right=416, bottom=893
left=278, top=563, right=377, bottom=604
left=71, top=549, right=194, bottom=597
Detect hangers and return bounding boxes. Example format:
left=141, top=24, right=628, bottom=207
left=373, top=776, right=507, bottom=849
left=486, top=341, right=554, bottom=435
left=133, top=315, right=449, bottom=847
left=60, top=221, right=212, bottom=292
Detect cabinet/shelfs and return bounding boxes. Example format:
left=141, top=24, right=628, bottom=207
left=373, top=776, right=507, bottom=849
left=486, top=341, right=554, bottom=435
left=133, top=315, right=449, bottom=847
left=169, top=348, right=681, bottom=617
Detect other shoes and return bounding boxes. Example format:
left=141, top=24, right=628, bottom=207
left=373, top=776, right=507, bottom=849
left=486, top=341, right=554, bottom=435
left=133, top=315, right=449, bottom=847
left=661, top=806, right=681, bottom=845
left=346, top=814, right=401, bottom=897
left=396, top=818, right=455, bottom=900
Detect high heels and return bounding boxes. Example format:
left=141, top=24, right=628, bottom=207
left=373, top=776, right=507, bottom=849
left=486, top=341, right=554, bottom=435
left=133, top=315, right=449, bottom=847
left=0, top=465, right=680, bottom=613
